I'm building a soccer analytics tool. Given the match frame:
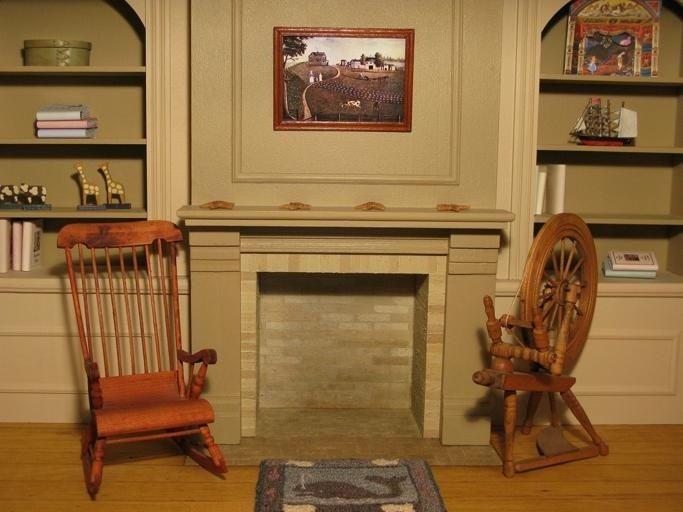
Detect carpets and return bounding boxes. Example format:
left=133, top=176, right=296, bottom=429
left=255, top=453, right=446, bottom=512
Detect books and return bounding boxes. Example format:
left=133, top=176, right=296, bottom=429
left=1, top=220, right=43, bottom=275
left=609, top=249, right=660, bottom=271
left=35, top=103, right=98, bottom=139
left=603, top=262, right=657, bottom=279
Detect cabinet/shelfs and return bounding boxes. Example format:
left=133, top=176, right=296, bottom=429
left=0, top=3, right=190, bottom=283
left=496, top=0, right=683, bottom=291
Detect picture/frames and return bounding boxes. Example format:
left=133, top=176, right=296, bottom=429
left=273, top=24, right=416, bottom=132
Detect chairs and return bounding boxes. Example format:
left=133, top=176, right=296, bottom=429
left=52, top=213, right=231, bottom=494
